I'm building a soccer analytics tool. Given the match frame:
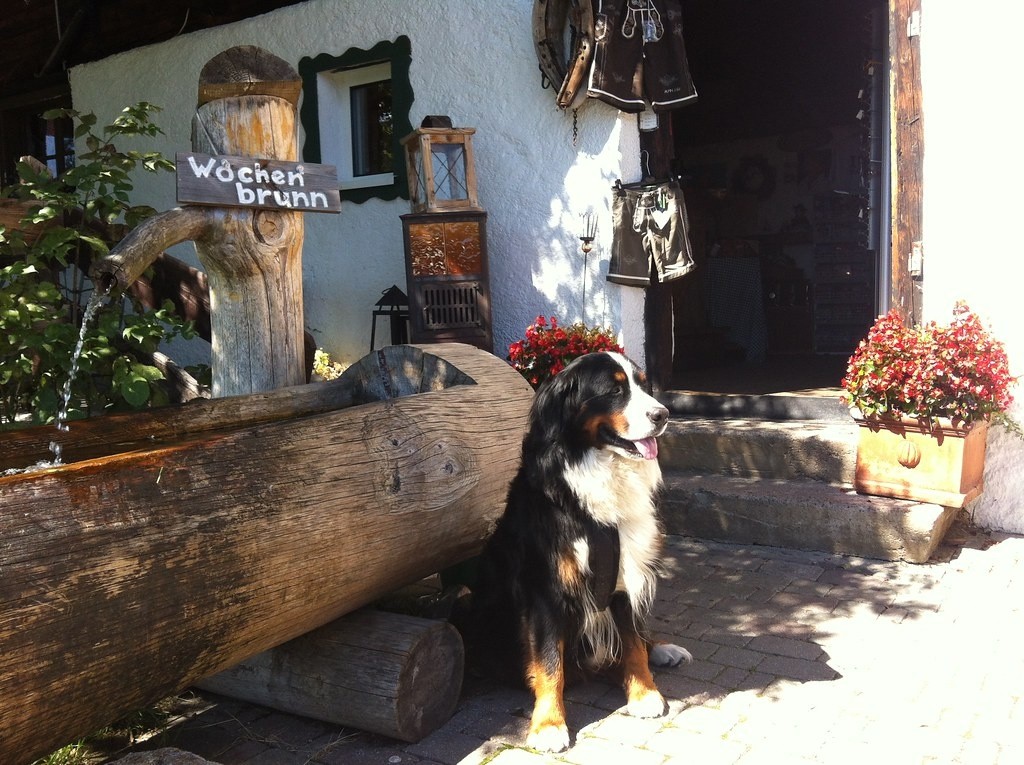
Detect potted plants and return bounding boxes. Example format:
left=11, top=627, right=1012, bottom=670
left=839, top=299, right=1024, bottom=508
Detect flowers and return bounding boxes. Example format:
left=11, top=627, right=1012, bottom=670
left=506, top=316, right=626, bottom=389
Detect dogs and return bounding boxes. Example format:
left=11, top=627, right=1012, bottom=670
left=373, top=351, right=694, bottom=753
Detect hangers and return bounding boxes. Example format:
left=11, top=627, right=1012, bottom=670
left=612, top=150, right=691, bottom=197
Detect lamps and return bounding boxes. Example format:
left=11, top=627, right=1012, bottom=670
left=370, top=284, right=411, bottom=351
